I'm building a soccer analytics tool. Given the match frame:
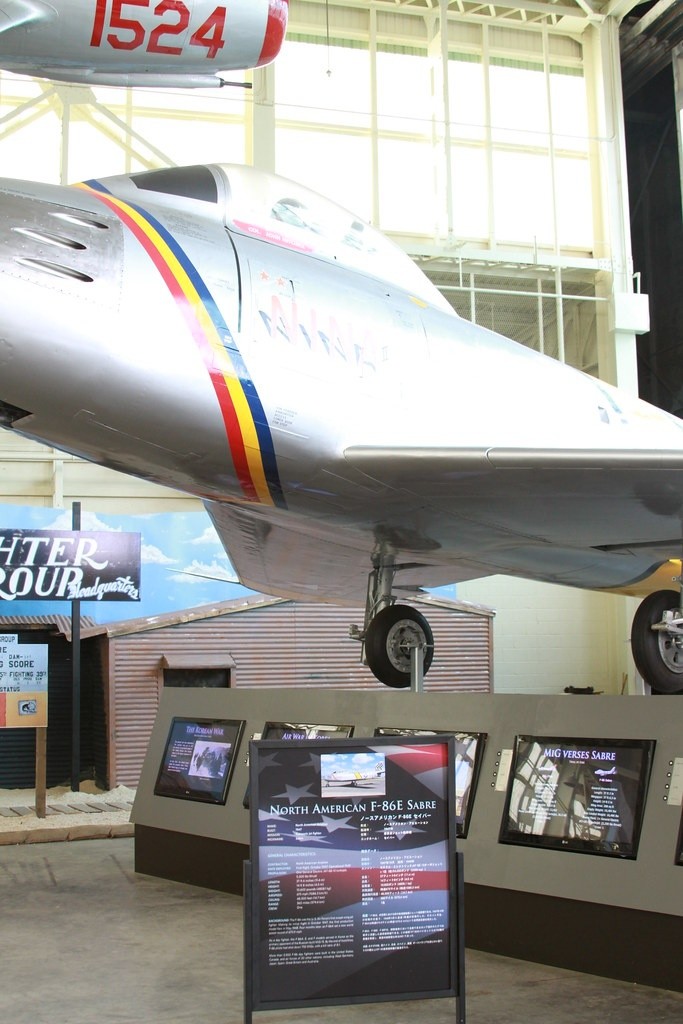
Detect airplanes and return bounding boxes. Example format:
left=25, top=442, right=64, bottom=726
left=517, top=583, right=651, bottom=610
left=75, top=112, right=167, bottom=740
left=324, top=762, right=384, bottom=789
left=1, top=158, right=683, bottom=694
